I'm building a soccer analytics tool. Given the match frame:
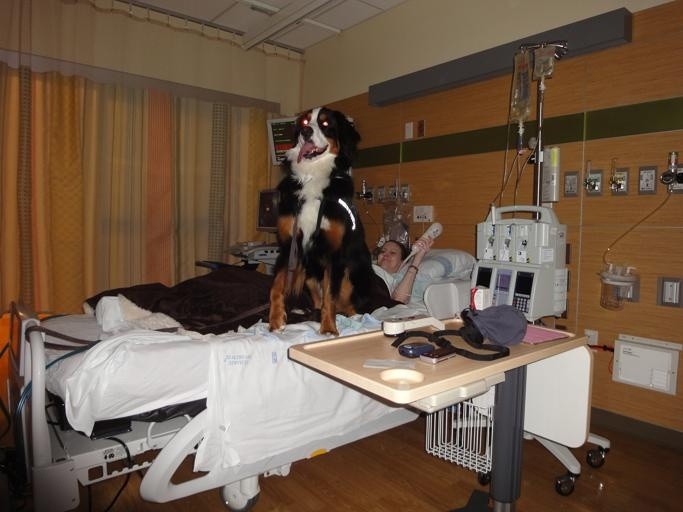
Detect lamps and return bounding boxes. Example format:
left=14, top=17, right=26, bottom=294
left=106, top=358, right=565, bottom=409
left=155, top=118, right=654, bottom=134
left=241, top=0, right=347, bottom=51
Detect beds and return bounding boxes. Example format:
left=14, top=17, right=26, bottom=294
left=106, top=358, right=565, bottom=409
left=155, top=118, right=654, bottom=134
left=8, top=279, right=472, bottom=512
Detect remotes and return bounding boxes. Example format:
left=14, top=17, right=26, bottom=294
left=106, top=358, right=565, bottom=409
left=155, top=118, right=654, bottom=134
left=400, top=341, right=433, bottom=358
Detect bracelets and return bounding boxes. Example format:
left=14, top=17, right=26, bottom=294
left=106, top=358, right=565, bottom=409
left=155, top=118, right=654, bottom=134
left=407, top=264, right=419, bottom=274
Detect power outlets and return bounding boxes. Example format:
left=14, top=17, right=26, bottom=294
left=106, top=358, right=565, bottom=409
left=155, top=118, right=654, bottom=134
left=362, top=184, right=410, bottom=204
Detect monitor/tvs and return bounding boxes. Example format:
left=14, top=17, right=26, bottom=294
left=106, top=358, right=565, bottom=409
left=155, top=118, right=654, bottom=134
left=266, top=117, right=300, bottom=166
left=256, top=187, right=280, bottom=232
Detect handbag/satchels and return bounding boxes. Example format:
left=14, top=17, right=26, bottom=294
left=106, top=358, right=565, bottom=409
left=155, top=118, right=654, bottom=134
left=461, top=304, right=528, bottom=346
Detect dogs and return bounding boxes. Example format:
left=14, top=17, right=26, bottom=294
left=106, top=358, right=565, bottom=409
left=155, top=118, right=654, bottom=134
left=268, top=107, right=406, bottom=336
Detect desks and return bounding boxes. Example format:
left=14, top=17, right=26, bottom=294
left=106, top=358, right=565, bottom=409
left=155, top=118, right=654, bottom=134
left=283, top=314, right=611, bottom=512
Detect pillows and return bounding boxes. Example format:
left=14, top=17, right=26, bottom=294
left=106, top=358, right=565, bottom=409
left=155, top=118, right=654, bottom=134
left=399, top=248, right=476, bottom=280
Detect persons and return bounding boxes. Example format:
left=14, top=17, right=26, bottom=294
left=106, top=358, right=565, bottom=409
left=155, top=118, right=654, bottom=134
left=370, top=235, right=434, bottom=305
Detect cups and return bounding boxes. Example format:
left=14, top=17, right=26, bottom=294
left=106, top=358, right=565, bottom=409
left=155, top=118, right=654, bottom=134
left=596, top=277, right=636, bottom=312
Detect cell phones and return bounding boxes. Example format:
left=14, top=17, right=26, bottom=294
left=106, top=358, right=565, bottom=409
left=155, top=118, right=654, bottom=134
left=421, top=345, right=456, bottom=365
